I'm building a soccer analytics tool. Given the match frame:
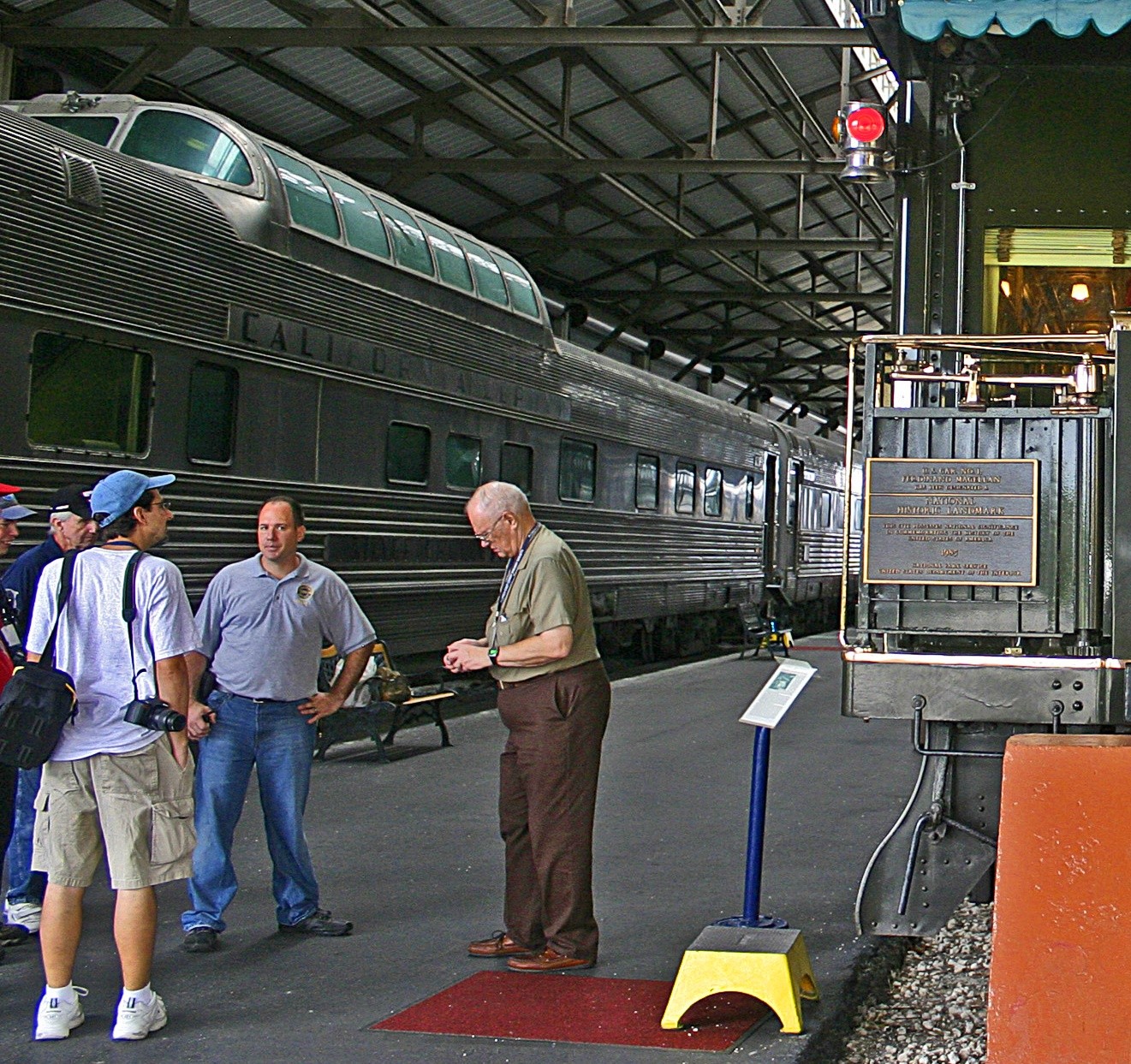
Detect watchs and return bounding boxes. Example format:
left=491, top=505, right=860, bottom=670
left=488, top=645, right=499, bottom=666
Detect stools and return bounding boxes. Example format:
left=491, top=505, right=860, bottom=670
left=661, top=927, right=820, bottom=1035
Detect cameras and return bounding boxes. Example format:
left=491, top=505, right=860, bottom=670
left=123, top=698, right=186, bottom=732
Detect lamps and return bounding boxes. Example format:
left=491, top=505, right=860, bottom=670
left=835, top=99, right=892, bottom=187
left=1072, top=281, right=1089, bottom=301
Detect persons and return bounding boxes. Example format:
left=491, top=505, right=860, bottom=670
left=1, top=489, right=98, bottom=933
left=26, top=470, right=205, bottom=1042
left=180, top=495, right=378, bottom=952
left=1, top=483, right=39, bottom=964
left=443, top=480, right=612, bottom=973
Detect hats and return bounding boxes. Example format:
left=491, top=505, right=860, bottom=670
left=0, top=493, right=38, bottom=519
left=91, top=470, right=176, bottom=527
left=49, top=488, right=93, bottom=518
left=0, top=483, right=22, bottom=492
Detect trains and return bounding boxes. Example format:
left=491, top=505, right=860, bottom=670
left=0, top=93, right=861, bottom=683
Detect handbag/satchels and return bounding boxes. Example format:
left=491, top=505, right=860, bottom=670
left=0, top=661, right=76, bottom=769
left=329, top=655, right=377, bottom=707
left=372, top=667, right=411, bottom=702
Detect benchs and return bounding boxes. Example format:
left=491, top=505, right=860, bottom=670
left=307, top=637, right=458, bottom=762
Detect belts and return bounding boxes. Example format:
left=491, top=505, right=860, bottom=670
left=496, top=679, right=529, bottom=690
left=238, top=694, right=285, bottom=704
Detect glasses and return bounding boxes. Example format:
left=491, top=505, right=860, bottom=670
left=151, top=502, right=171, bottom=510
left=474, top=515, right=503, bottom=541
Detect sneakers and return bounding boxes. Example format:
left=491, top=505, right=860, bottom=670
left=278, top=908, right=353, bottom=934
left=35, top=986, right=89, bottom=1039
left=183, top=926, right=217, bottom=951
left=112, top=991, right=167, bottom=1039
left=3, top=896, right=43, bottom=933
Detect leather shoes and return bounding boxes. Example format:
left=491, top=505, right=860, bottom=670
left=467, top=930, right=545, bottom=956
left=506, top=946, right=593, bottom=972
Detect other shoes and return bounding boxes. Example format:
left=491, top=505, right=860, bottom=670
left=0, top=923, right=28, bottom=945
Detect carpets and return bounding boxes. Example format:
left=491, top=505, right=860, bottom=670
left=360, top=970, right=776, bottom=1054
